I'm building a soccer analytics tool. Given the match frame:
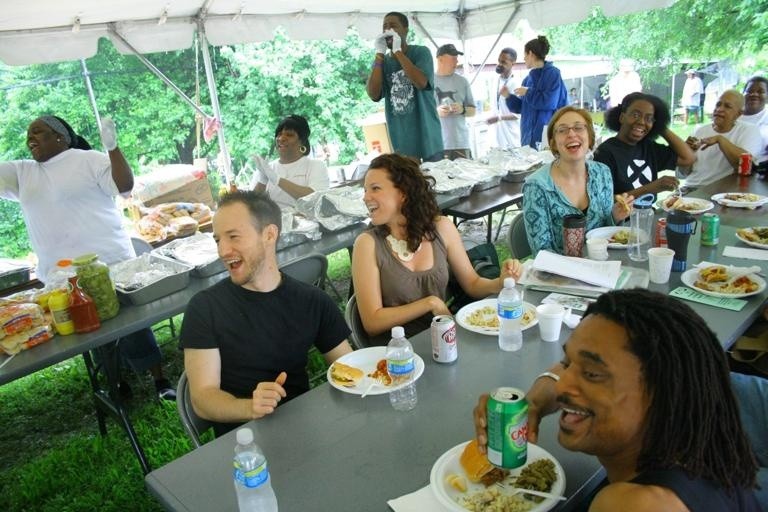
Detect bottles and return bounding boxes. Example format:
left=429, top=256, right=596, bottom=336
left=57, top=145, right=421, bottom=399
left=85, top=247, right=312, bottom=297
left=561, top=213, right=586, bottom=258
left=496, top=277, right=524, bottom=353
left=47, top=252, right=121, bottom=337
left=665, top=208, right=697, bottom=273
left=231, top=426, right=280, bottom=511
left=627, top=192, right=657, bottom=263
left=384, top=324, right=419, bottom=414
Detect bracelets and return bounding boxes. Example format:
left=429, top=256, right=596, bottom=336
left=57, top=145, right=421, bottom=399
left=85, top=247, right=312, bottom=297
left=373, top=55, right=383, bottom=67
left=533, top=372, right=559, bottom=384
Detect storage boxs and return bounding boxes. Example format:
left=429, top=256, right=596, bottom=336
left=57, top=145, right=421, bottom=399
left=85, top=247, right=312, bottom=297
left=142, top=158, right=215, bottom=210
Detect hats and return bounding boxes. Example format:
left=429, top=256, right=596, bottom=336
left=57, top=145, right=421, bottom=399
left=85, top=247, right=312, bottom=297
left=437, top=44, right=464, bottom=56
left=286, top=113, right=310, bottom=156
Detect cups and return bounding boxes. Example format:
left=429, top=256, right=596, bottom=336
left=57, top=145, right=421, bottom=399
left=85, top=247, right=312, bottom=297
left=586, top=237, right=610, bottom=262
left=535, top=302, right=566, bottom=343
left=647, top=246, right=676, bottom=285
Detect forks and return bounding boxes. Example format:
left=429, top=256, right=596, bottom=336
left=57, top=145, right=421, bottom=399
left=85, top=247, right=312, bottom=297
left=494, top=482, right=567, bottom=503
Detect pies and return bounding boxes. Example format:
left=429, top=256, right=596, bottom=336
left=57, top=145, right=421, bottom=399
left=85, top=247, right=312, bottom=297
left=330, top=362, right=364, bottom=386
left=460, top=438, right=495, bottom=484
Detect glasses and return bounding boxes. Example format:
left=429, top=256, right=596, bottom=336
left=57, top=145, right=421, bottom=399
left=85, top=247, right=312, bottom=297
left=554, top=124, right=590, bottom=135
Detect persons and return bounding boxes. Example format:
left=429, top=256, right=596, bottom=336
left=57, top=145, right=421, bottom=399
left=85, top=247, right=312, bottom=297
left=352, top=154, right=523, bottom=346
left=178, top=191, right=354, bottom=439
left=522, top=77, right=768, bottom=258
left=729, top=371, right=768, bottom=511
left=366, top=12, right=444, bottom=162
left=495, top=37, right=568, bottom=151
left=254, top=115, right=327, bottom=285
left=434, top=44, right=475, bottom=160
left=474, top=288, right=761, bottom=512
left=0, top=115, right=177, bottom=400
left=682, top=69, right=705, bottom=124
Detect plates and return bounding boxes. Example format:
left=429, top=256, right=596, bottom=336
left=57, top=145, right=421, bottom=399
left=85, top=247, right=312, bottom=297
left=679, top=265, right=768, bottom=299
left=656, top=197, right=716, bottom=215
left=428, top=435, right=568, bottom=512
left=710, top=191, right=768, bottom=208
left=584, top=225, right=650, bottom=251
left=326, top=344, right=426, bottom=396
left=734, top=225, right=768, bottom=249
left=455, top=298, right=540, bottom=336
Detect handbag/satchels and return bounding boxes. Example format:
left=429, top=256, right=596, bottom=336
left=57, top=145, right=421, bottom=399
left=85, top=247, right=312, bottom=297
left=447, top=243, right=500, bottom=305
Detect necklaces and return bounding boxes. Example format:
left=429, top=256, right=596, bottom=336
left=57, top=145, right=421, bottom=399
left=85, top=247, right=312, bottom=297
left=385, top=235, right=422, bottom=262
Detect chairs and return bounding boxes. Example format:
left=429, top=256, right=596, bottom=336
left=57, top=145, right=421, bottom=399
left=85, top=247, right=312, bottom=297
left=129, top=237, right=179, bottom=351
left=177, top=370, right=213, bottom=448
left=277, top=252, right=344, bottom=384
left=508, top=212, right=532, bottom=260
left=459, top=238, right=480, bottom=250
left=344, top=291, right=371, bottom=348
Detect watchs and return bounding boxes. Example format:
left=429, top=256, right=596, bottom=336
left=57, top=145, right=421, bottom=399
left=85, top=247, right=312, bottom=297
left=461, top=105, right=467, bottom=114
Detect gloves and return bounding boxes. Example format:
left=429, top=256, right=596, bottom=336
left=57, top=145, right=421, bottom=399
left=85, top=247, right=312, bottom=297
left=390, top=29, right=403, bottom=53
left=100, top=119, right=118, bottom=151
left=374, top=32, right=388, bottom=56
left=256, top=155, right=281, bottom=188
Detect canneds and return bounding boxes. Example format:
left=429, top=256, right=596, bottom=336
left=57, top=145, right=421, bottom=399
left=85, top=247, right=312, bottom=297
left=701, top=212, right=720, bottom=246
left=656, top=217, right=668, bottom=247
left=430, top=315, right=458, bottom=363
left=738, top=153, right=752, bottom=175
left=71, top=254, right=119, bottom=322
left=485, top=386, right=529, bottom=470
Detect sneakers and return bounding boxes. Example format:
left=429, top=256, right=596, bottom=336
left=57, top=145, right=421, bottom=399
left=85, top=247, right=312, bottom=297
left=154, top=379, right=177, bottom=399
